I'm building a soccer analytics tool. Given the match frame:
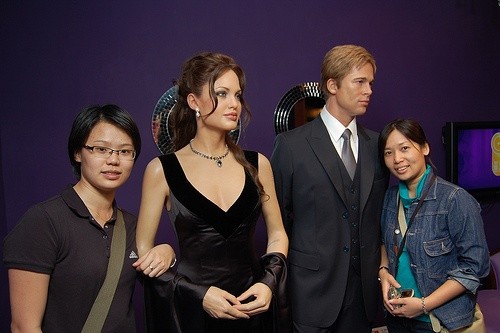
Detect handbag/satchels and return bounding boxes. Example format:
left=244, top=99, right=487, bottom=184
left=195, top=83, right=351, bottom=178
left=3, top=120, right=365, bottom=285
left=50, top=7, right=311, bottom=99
left=429, top=302, right=486, bottom=333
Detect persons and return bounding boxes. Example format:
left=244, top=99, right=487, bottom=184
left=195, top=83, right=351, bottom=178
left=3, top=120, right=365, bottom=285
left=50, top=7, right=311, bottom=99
left=0, top=104, right=176, bottom=333
left=136, top=52, right=289, bottom=333
left=379, top=118, right=490, bottom=333
left=270, top=45, right=391, bottom=333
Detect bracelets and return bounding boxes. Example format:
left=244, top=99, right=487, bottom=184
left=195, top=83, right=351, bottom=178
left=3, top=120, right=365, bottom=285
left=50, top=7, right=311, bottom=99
left=379, top=266, right=389, bottom=271
left=170, top=258, right=176, bottom=267
left=422, top=297, right=428, bottom=315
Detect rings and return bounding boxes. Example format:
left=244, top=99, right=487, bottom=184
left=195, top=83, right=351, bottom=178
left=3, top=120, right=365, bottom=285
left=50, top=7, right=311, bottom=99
left=149, top=265, right=154, bottom=270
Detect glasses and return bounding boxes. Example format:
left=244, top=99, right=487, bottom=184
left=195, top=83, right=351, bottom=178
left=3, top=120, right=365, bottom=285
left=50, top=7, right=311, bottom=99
left=83, top=145, right=136, bottom=160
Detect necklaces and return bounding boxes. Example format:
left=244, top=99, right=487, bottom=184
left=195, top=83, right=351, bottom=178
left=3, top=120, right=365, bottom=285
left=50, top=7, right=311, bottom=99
left=190, top=139, right=229, bottom=167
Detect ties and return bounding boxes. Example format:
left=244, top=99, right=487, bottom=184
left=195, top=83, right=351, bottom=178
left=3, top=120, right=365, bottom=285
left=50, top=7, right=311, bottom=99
left=341, top=128, right=357, bottom=180
